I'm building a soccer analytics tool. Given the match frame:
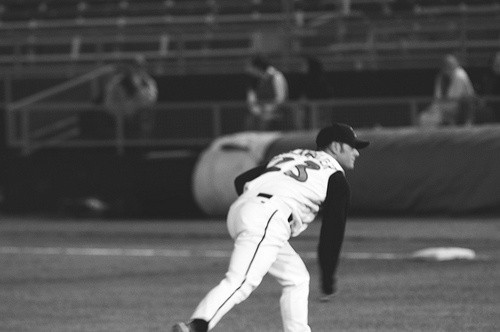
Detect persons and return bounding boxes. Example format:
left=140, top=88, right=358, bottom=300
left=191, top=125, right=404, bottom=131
left=241, top=53, right=289, bottom=131
left=289, top=58, right=333, bottom=129
left=172, top=122, right=370, bottom=331
left=418, top=54, right=479, bottom=126
left=104, top=54, right=157, bottom=139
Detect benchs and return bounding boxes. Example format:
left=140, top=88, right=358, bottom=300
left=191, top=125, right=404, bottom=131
left=0, top=0, right=499, bottom=162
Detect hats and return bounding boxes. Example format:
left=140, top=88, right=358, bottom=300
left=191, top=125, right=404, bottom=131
left=316, top=123, right=370, bottom=150
